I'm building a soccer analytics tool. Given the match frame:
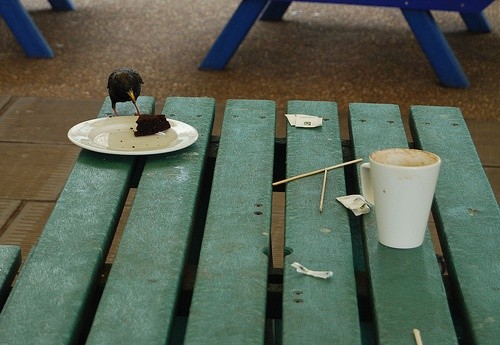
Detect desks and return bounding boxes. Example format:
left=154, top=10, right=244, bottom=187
left=0, top=95, right=500, bottom=345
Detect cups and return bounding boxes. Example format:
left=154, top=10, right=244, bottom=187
left=360, top=148, right=441, bottom=249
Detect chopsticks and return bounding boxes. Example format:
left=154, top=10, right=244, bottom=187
left=271, top=158, right=363, bottom=212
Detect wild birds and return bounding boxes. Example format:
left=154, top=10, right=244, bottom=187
left=107, top=67, right=145, bottom=116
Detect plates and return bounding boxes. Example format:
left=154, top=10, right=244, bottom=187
left=67, top=116, right=198, bottom=155
